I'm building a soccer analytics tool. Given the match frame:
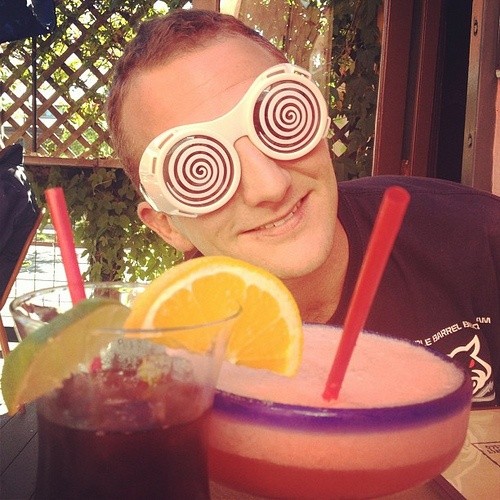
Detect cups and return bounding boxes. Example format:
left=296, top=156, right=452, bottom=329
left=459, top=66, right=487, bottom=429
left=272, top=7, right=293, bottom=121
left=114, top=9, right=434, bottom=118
left=9, top=281, right=242, bottom=500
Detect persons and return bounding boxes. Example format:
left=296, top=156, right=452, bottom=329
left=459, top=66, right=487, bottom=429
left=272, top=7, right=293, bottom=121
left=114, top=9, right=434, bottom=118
left=104, top=9, right=500, bottom=410
left=1, top=139, right=40, bottom=302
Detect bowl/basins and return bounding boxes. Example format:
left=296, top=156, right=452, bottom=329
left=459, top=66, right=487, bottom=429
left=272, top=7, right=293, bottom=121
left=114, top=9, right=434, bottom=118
left=203, top=320, right=473, bottom=500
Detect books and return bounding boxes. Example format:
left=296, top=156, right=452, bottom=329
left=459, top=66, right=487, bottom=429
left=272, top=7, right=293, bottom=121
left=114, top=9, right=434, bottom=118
left=431, top=409, right=499, bottom=500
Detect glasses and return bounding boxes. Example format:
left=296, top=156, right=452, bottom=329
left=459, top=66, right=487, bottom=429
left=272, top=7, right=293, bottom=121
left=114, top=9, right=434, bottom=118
left=134, top=63, right=332, bottom=218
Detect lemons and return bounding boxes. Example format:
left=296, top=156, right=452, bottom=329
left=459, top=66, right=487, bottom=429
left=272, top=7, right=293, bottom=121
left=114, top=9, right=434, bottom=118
left=123, top=256, right=302, bottom=377
left=1, top=297, right=128, bottom=415
